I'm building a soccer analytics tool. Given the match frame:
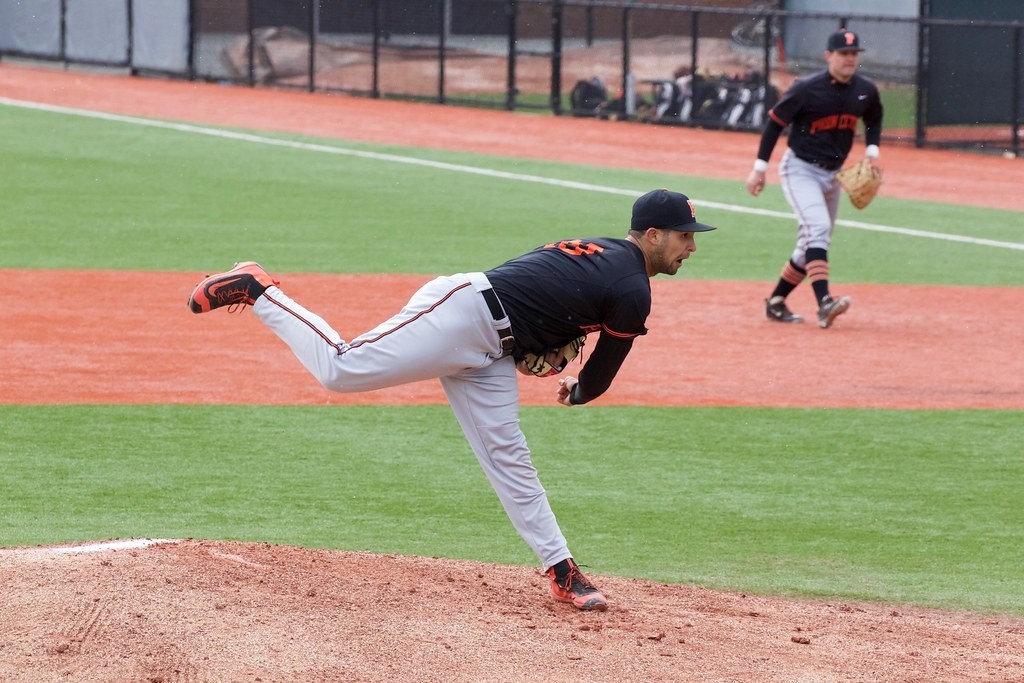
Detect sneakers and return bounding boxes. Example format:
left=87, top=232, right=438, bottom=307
left=766, top=295, right=804, bottom=323
left=817, top=294, right=850, bottom=328
left=548, top=558, right=610, bottom=612
left=188, top=261, right=280, bottom=314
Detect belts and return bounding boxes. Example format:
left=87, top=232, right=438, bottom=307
left=796, top=154, right=841, bottom=170
left=481, top=288, right=516, bottom=355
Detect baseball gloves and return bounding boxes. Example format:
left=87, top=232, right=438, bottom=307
left=834, top=158, right=884, bottom=212
left=523, top=331, right=586, bottom=377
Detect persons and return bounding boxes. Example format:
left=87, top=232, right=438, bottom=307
left=189, top=188, right=717, bottom=613
left=747, top=29, right=884, bottom=327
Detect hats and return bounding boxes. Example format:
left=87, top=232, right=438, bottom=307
left=826, top=28, right=865, bottom=52
left=631, top=188, right=717, bottom=232
